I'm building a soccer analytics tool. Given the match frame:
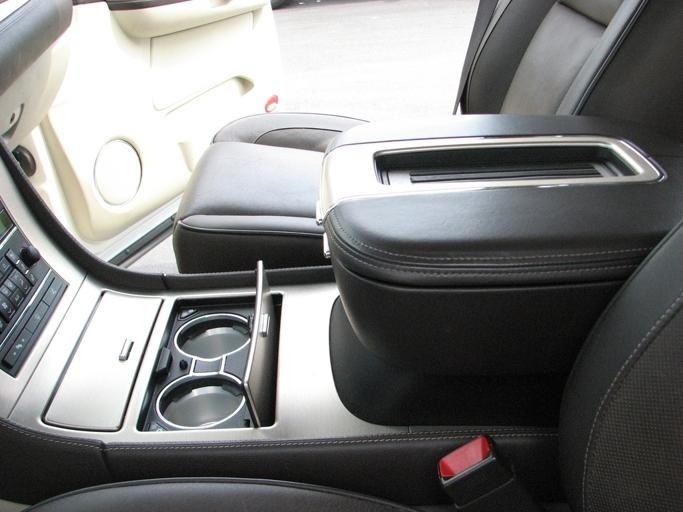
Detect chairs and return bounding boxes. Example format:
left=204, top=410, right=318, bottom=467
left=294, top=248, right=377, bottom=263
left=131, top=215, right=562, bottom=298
left=168, top=0, right=683, bottom=273
left=15, top=212, right=683, bottom=512
